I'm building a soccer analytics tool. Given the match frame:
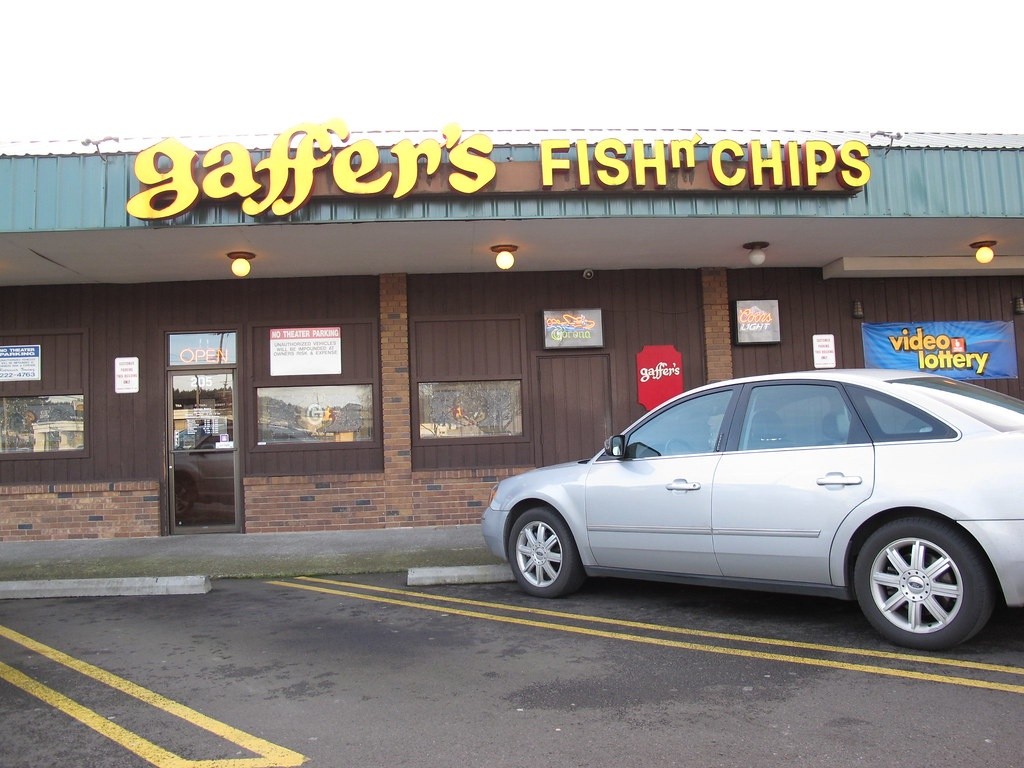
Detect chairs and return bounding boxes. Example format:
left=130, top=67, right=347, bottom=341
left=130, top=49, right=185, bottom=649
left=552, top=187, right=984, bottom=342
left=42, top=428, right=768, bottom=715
left=749, top=411, right=795, bottom=450
left=814, top=410, right=848, bottom=446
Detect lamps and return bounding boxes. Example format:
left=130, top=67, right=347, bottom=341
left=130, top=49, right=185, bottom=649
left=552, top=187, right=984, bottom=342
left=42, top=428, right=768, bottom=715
left=742, top=242, right=771, bottom=266
left=1014, top=297, right=1024, bottom=314
left=491, top=244, right=518, bottom=270
left=854, top=300, right=863, bottom=320
left=226, top=252, right=257, bottom=278
left=969, top=240, right=998, bottom=264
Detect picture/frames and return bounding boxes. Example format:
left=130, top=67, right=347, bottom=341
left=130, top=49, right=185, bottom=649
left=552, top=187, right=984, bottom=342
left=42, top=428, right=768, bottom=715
left=734, top=297, right=781, bottom=345
left=541, top=308, right=605, bottom=350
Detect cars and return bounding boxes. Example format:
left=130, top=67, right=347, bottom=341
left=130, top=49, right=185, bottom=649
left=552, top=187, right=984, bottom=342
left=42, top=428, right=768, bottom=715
left=175, top=427, right=201, bottom=449
left=172, top=424, right=318, bottom=516
left=482, top=369, right=1024, bottom=655
